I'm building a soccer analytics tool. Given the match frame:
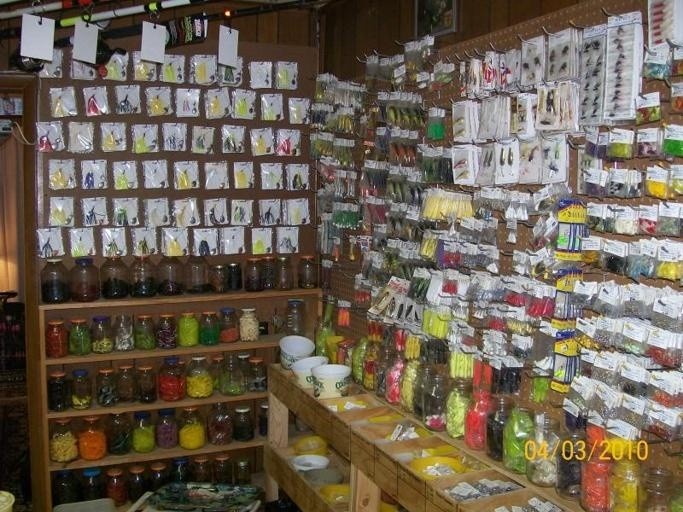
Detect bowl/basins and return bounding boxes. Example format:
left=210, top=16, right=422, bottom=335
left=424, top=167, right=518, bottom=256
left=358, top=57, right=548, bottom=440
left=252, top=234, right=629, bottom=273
left=293, top=435, right=328, bottom=458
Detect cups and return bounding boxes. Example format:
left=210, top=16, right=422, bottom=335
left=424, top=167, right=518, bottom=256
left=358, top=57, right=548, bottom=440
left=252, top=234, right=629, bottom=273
left=276, top=335, right=328, bottom=390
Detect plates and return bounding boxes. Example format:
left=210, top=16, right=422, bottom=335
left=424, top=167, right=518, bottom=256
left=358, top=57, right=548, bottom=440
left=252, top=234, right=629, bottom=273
left=292, top=455, right=330, bottom=470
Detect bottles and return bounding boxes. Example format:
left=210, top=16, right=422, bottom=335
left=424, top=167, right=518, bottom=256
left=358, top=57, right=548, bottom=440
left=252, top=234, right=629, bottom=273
left=40, top=250, right=319, bottom=357
left=311, top=321, right=683, bottom=512
left=47, top=357, right=266, bottom=504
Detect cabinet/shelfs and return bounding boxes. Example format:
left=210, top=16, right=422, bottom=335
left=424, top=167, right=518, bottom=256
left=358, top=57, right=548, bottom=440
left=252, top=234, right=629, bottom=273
left=38, top=287, right=323, bottom=511
left=262, top=356, right=583, bottom=510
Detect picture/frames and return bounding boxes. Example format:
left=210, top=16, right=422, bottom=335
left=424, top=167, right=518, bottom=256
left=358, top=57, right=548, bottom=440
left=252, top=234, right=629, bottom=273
left=413, top=1, right=458, bottom=42
